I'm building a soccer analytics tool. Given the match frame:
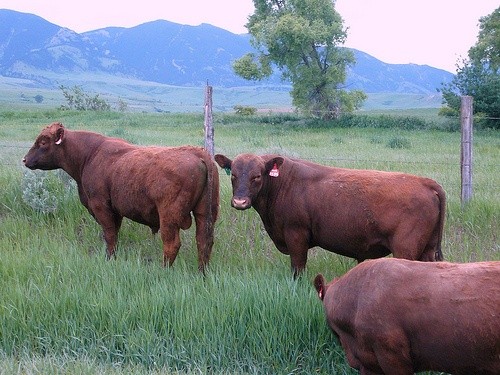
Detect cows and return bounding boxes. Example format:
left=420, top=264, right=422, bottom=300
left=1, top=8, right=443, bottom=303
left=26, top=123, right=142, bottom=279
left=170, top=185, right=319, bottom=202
left=23, top=122, right=218, bottom=275
left=313, top=258, right=500, bottom=375
left=214, top=154, right=446, bottom=279
